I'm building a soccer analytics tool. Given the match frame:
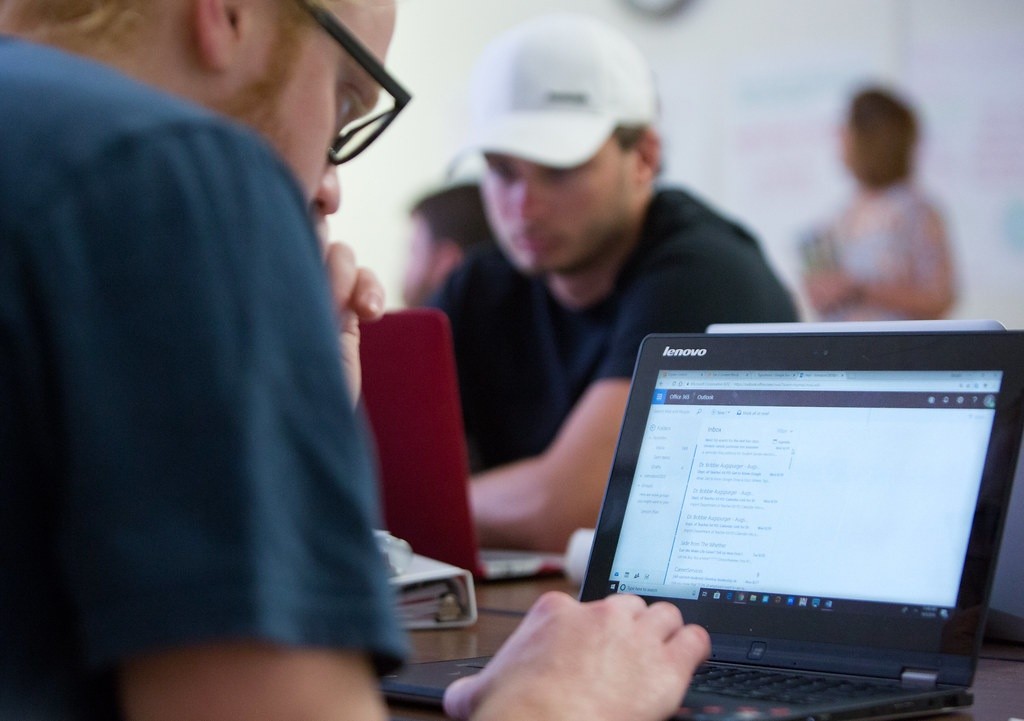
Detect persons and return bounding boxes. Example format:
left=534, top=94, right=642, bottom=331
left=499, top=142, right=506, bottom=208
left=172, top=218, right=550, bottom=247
left=392, top=17, right=957, bottom=555
left=0, top=0, right=712, bottom=721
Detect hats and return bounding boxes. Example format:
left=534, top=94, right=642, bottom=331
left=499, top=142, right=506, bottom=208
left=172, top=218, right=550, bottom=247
left=444, top=13, right=659, bottom=178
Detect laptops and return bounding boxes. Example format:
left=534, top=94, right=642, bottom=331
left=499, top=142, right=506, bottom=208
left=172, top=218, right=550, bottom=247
left=357, top=304, right=1024, bottom=721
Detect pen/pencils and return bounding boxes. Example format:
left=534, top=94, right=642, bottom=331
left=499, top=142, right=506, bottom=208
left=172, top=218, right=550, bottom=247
left=483, top=559, right=561, bottom=580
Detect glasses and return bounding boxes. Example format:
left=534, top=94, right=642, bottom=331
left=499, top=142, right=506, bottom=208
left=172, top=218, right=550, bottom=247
left=299, top=0, right=411, bottom=165
